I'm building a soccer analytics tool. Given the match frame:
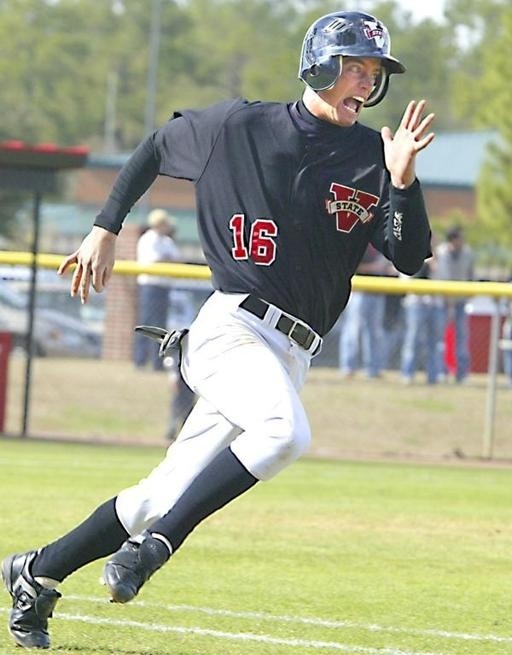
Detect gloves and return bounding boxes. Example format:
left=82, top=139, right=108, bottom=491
left=135, top=325, right=187, bottom=357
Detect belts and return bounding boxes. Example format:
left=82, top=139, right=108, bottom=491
left=238, top=294, right=321, bottom=356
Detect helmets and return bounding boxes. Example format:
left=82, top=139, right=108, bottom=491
left=297, top=10, right=407, bottom=107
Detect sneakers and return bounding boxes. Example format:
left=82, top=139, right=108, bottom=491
left=2, top=550, right=61, bottom=649
left=105, top=529, right=170, bottom=603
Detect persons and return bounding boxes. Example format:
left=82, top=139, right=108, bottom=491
left=3, top=10, right=433, bottom=647
left=343, top=225, right=511, bottom=388
left=132, top=208, right=182, bottom=372
